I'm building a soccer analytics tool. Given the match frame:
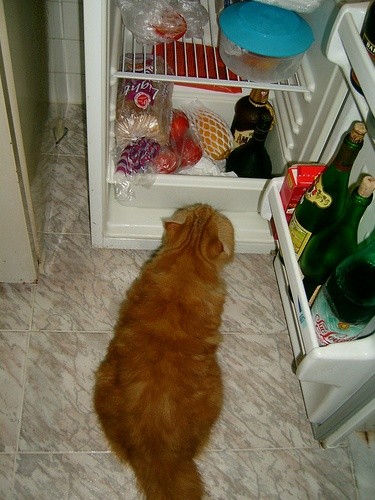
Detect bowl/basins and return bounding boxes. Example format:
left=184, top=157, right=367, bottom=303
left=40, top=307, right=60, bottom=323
left=217, top=1, right=316, bottom=83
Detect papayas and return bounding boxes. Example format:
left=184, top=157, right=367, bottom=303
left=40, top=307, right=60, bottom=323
left=197, top=114, right=232, bottom=161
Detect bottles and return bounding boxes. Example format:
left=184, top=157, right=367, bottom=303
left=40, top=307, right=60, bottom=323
left=289, top=120, right=368, bottom=262
left=311, top=228, right=375, bottom=354
left=297, top=174, right=375, bottom=306
left=230, top=89, right=274, bottom=141
left=225, top=112, right=272, bottom=181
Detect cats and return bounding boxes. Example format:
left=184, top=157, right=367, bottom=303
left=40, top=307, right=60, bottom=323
left=93, top=203, right=236, bottom=500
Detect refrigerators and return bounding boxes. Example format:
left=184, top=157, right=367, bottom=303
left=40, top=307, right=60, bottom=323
left=82, top=1, right=375, bottom=450
